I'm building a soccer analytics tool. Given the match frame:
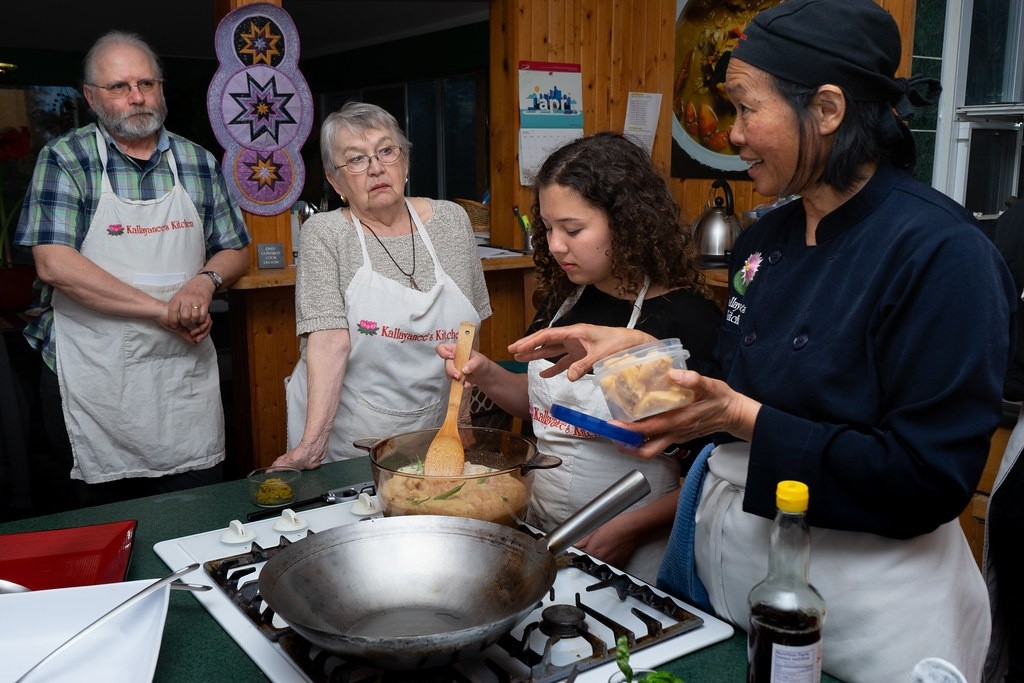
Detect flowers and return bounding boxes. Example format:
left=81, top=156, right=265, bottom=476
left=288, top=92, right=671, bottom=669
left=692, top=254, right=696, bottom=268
left=0, top=125, right=34, bottom=178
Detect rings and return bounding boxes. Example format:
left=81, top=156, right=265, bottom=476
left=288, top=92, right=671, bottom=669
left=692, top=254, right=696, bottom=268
left=192, top=306, right=199, bottom=309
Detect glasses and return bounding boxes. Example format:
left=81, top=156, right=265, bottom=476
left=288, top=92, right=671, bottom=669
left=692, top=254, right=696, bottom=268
left=88, top=78, right=166, bottom=98
left=334, top=142, right=404, bottom=173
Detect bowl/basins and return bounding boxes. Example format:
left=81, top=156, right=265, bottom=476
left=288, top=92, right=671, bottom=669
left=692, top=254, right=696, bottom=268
left=248, top=466, right=303, bottom=507
left=592, top=337, right=695, bottom=422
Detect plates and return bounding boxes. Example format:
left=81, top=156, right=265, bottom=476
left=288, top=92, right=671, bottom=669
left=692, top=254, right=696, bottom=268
left=0, top=519, right=139, bottom=596
left=0, top=579, right=171, bottom=683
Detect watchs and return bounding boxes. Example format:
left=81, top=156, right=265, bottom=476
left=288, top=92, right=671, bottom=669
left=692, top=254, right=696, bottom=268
left=199, top=270, right=223, bottom=288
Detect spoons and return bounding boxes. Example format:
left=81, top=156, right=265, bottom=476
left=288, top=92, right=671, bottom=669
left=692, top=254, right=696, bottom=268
left=299, top=202, right=318, bottom=226
left=0, top=576, right=212, bottom=596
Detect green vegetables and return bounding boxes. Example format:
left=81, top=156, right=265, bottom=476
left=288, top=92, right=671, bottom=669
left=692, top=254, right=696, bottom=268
left=615, top=635, right=684, bottom=683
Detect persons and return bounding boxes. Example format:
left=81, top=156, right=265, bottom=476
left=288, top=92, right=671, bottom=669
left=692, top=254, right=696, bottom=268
left=435, top=131, right=724, bottom=590
left=267, top=102, right=492, bottom=471
left=981, top=199, right=1024, bottom=683
left=13, top=32, right=254, bottom=510
left=506, top=0, right=1017, bottom=683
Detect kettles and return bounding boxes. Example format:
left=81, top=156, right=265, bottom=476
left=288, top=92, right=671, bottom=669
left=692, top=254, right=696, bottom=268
left=687, top=177, right=744, bottom=266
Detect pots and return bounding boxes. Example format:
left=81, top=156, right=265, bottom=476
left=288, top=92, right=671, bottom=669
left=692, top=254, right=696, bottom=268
left=352, top=428, right=562, bottom=531
left=256, top=470, right=651, bottom=668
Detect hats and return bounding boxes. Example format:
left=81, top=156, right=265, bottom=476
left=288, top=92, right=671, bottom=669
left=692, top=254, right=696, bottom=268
left=731, top=0, right=942, bottom=123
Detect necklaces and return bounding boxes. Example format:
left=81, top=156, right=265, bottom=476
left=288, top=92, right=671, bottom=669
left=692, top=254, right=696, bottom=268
left=360, top=202, right=421, bottom=292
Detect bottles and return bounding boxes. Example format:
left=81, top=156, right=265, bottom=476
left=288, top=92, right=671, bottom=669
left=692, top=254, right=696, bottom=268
left=743, top=480, right=828, bottom=683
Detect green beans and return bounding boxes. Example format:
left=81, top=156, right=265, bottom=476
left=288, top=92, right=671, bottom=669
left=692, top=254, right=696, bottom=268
left=407, top=453, right=505, bottom=504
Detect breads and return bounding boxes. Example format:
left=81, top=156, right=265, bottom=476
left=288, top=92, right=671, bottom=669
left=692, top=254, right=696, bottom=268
left=600, top=350, right=694, bottom=417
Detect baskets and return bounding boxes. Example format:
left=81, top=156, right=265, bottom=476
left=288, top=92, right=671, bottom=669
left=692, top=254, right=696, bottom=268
left=455, top=198, right=491, bottom=231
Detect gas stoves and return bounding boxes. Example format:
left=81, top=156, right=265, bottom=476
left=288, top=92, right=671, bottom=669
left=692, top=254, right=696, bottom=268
left=154, top=492, right=735, bottom=683
